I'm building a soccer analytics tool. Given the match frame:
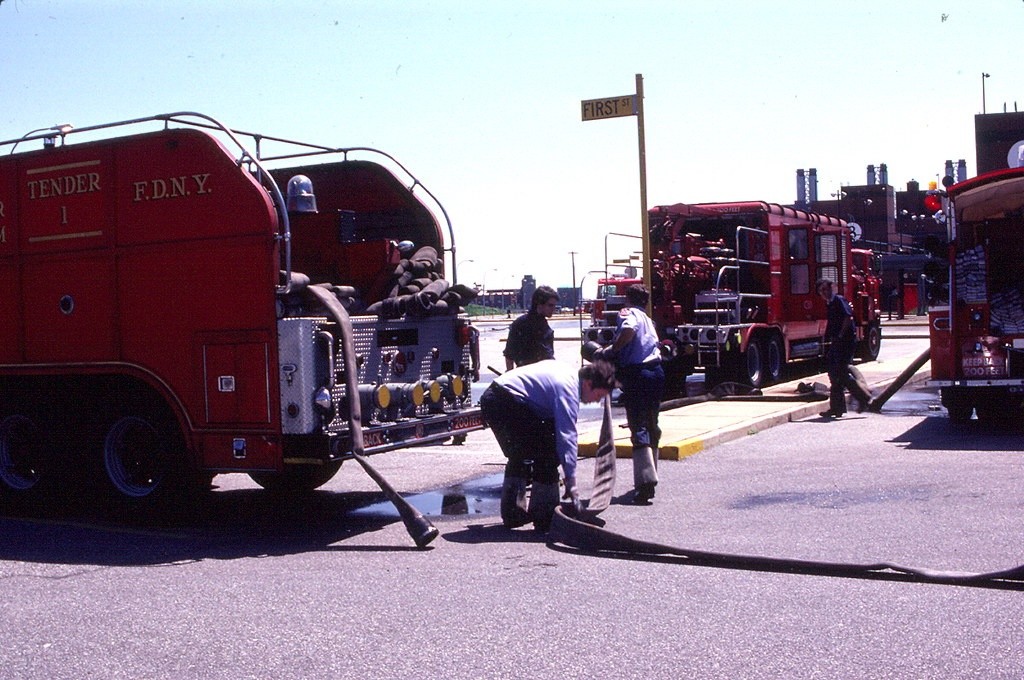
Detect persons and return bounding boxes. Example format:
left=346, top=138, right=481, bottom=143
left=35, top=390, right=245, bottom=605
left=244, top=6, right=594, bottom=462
left=503, top=287, right=560, bottom=371
left=581, top=283, right=666, bottom=499
left=814, top=278, right=872, bottom=417
left=481, top=357, right=617, bottom=535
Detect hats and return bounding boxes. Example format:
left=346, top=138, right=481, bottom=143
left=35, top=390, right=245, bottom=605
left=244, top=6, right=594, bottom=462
left=815, top=276, right=834, bottom=293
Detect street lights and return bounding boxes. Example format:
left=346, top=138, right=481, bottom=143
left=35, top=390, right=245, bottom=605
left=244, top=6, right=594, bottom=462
left=831, top=189, right=848, bottom=219
left=897, top=208, right=925, bottom=320
left=862, top=199, right=873, bottom=249
left=568, top=251, right=579, bottom=316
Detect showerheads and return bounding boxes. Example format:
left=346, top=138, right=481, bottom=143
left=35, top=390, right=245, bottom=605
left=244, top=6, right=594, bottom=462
left=581, top=341, right=602, bottom=362
left=406, top=515, right=440, bottom=548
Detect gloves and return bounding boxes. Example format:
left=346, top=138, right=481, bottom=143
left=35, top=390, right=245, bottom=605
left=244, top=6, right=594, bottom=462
left=602, top=344, right=618, bottom=360
left=592, top=347, right=603, bottom=359
left=562, top=478, right=579, bottom=505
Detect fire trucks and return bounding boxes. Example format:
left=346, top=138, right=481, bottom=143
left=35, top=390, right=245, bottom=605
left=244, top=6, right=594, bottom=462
left=0, top=111, right=501, bottom=547
left=580, top=200, right=882, bottom=389
left=924, top=166, right=1024, bottom=418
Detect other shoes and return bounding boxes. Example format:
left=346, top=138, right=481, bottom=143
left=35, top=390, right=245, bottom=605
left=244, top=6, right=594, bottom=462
left=634, top=486, right=655, bottom=498
left=501, top=510, right=533, bottom=529
left=819, top=409, right=842, bottom=417
left=533, top=513, right=552, bottom=533
left=855, top=394, right=871, bottom=414
